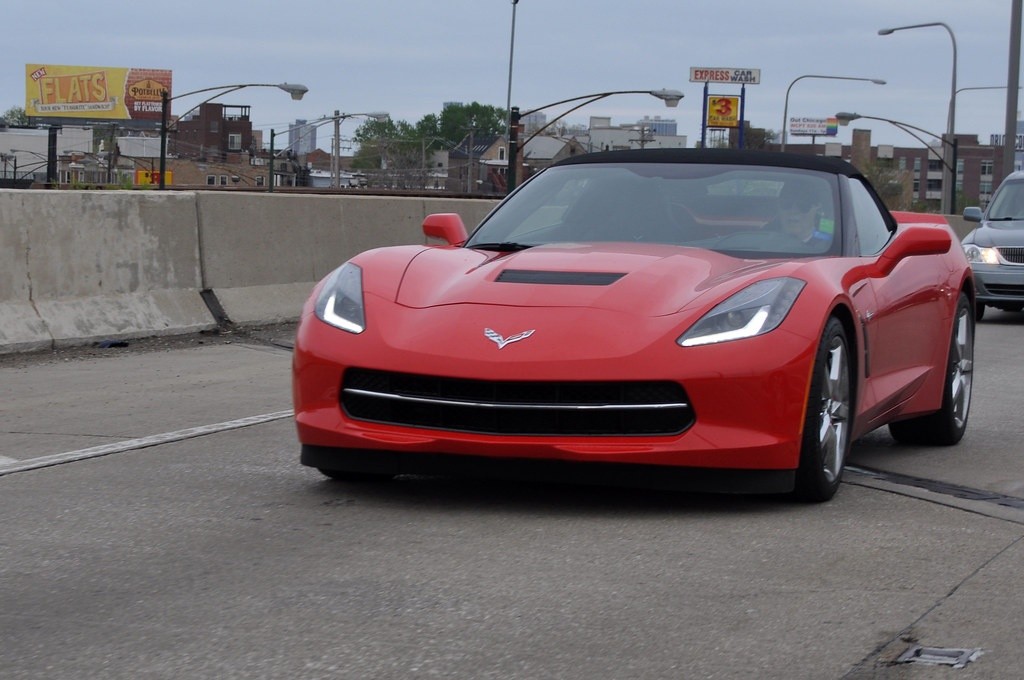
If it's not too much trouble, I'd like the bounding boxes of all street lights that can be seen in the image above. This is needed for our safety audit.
[832,111,958,214]
[158,83,310,190]
[876,21,957,135]
[504,87,685,196]
[267,111,390,193]
[781,74,888,153]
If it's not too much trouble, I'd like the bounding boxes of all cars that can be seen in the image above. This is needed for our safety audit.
[959,171,1024,321]
[290,146,979,505]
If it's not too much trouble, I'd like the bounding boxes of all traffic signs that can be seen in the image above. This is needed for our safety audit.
[688,66,761,85]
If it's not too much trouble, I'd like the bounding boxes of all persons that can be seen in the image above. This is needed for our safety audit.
[760,175,834,256]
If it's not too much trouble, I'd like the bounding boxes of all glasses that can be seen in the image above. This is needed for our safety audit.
[778,199,813,209]
